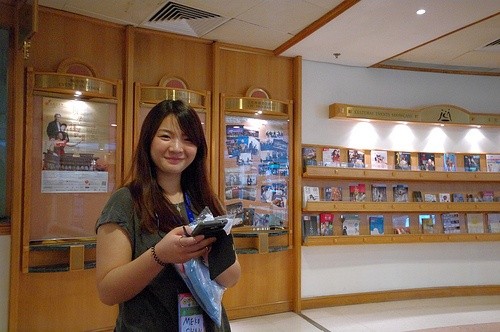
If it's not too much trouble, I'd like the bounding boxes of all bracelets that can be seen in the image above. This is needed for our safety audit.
[164,191,183,218]
[152,244,165,266]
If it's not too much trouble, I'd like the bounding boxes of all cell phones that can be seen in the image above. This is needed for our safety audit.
[192,219,228,241]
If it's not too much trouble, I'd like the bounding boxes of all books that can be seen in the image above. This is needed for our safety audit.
[302,148,316,167]
[348,150,365,169]
[441,213,500,234]
[486,155,500,172]
[418,152,435,171]
[443,153,456,172]
[453,191,494,202]
[371,151,387,169]
[391,214,436,235]
[371,184,450,203]
[322,148,341,167]
[342,214,384,235]
[303,213,333,236]
[464,155,480,172]
[303,183,367,208]
[394,151,410,171]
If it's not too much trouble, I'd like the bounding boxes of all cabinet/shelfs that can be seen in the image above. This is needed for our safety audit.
[301,145,500,247]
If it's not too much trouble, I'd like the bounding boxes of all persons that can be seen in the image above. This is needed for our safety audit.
[46,114,69,170]
[226,125,288,226]
[95,99,241,332]
